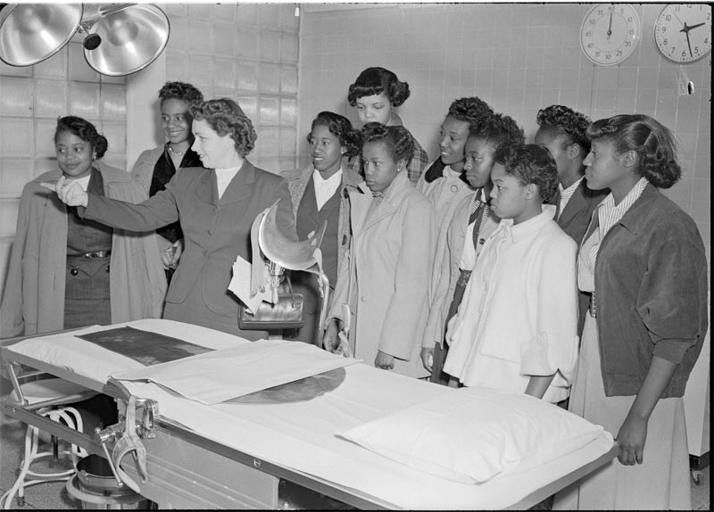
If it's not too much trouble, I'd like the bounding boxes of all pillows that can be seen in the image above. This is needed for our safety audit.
[333,385,606,487]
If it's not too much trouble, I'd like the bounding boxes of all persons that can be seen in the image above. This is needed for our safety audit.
[0,114,167,342]
[128,80,202,294]
[39,98,296,342]
[550,114,708,511]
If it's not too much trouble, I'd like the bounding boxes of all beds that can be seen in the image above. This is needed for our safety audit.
[0,317,619,510]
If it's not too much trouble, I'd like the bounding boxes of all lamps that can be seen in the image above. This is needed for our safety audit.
[0,4,173,80]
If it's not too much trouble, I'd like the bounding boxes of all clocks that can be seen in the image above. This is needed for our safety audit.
[576,3,643,68]
[651,2,713,64]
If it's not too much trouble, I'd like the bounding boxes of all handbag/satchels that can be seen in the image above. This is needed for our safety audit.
[234,292,306,331]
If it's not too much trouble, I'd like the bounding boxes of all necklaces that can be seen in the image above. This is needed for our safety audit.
[167,145,191,156]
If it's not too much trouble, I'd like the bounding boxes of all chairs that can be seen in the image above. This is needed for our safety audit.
[0,335,103,504]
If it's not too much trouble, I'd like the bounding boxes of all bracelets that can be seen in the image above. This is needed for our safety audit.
[81,190,86,206]
